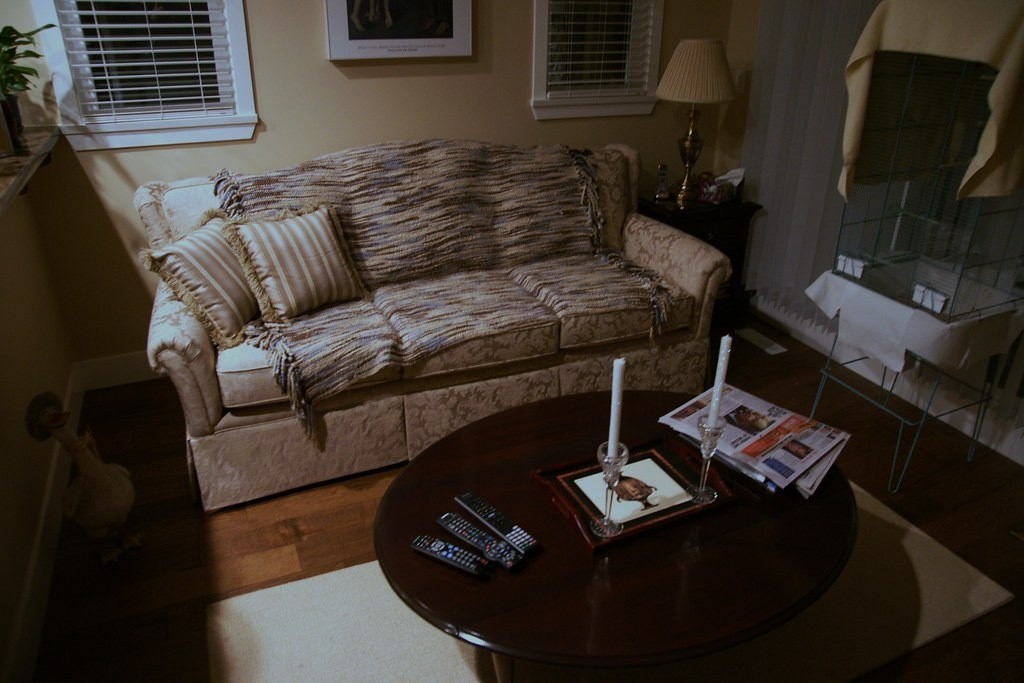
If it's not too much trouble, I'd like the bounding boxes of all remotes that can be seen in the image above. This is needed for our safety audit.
[454,488,540,555]
[436,511,524,572]
[409,534,490,575]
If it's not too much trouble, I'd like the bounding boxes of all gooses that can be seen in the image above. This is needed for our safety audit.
[37,403,142,564]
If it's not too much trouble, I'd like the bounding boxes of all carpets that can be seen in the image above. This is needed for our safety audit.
[206,479,1014,683]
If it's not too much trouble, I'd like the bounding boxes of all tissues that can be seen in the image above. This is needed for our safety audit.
[695,167,747,204]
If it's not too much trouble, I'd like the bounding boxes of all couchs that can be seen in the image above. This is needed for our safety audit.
[133,138,729,513]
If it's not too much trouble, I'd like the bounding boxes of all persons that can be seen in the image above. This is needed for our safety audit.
[350,0,451,35]
[724,412,770,431]
[784,440,809,458]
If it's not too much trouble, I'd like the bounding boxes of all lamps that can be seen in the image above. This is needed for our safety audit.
[654,37,737,209]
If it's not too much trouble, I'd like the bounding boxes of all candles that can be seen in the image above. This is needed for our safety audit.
[607,357,627,457]
[709,335,732,427]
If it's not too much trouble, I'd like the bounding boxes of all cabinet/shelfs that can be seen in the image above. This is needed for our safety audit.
[639,192,763,316]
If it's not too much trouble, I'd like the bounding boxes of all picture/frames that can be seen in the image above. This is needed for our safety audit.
[324,0,473,61]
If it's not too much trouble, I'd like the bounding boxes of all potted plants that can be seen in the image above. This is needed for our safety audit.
[0,23,55,159]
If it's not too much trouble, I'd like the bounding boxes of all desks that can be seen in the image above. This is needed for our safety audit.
[804,258,1024,496]
[0,126,59,215]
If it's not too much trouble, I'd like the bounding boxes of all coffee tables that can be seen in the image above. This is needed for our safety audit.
[372,390,859,683]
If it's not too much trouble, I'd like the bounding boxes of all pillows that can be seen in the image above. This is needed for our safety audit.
[137,208,261,350]
[222,202,369,326]
